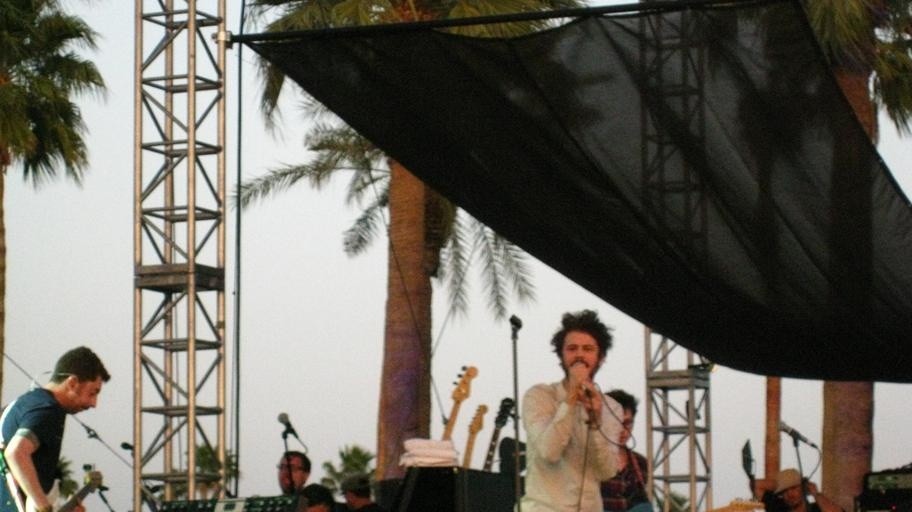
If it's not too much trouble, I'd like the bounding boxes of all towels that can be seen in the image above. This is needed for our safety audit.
[399,439,458,468]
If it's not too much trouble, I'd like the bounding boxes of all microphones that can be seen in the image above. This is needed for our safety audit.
[776,420,818,449]
[278,413,299,439]
[121,443,133,450]
[571,359,591,400]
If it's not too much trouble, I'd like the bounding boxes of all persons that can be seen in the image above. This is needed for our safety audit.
[298,483,337,512]
[339,472,380,511]
[276,450,311,494]
[512,309,625,511]
[748,469,848,511]
[603,388,654,511]
[1,346,113,512]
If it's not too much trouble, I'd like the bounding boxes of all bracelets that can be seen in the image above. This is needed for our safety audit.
[814,492,822,499]
[35,504,53,512]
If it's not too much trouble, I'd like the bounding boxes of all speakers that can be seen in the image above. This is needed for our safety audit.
[388,467,526,512]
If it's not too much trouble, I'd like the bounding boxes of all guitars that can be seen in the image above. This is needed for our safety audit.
[481,397,515,473]
[26,472,104,512]
[443,365,477,442]
[463,405,489,470]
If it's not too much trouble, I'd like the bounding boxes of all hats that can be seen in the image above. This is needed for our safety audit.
[775,468,801,494]
